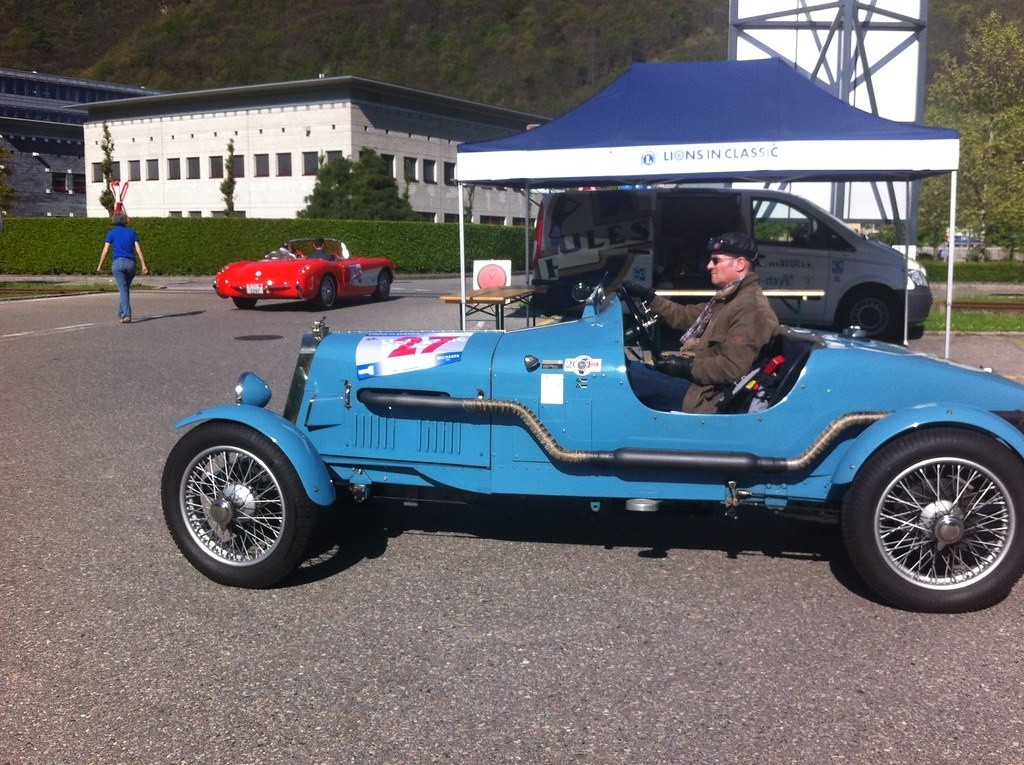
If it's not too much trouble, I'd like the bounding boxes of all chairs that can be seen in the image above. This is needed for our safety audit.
[715,324,816,414]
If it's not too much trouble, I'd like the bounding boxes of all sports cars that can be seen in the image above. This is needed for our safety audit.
[213,237,395,309]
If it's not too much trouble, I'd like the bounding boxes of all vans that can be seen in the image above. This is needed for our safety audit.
[532,187,934,340]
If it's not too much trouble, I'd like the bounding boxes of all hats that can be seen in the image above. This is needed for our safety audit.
[708,232,761,267]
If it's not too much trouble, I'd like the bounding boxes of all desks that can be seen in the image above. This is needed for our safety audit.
[440,285,536,330]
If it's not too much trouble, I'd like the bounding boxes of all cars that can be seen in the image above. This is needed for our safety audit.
[946,236,982,248]
[159,271,1024,613]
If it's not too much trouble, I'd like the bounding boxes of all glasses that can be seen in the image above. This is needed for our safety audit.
[707,257,736,266]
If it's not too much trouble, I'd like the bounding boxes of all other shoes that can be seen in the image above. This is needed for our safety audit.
[119,316,131,323]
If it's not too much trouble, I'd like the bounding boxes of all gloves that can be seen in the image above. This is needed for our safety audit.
[651,355,694,378]
[618,280,655,307]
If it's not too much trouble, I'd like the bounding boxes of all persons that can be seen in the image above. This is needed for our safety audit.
[282,238,338,260]
[622,233,778,414]
[97,212,149,323]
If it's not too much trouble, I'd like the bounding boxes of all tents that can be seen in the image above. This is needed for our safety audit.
[947,235,981,250]
[456,56,961,359]
